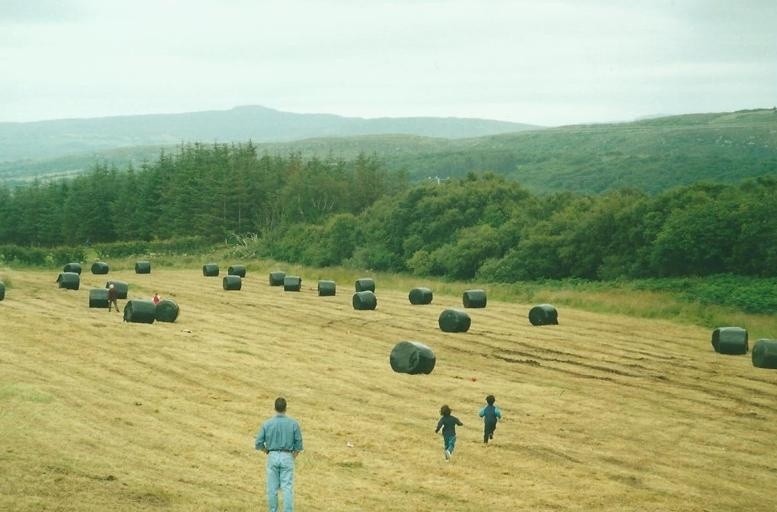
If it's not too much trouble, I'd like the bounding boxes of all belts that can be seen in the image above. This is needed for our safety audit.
[267,449,294,454]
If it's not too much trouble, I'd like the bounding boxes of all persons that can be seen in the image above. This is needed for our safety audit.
[434,405,463,462]
[253,397,304,511]
[479,394,501,444]
[108,284,119,312]
[152,292,159,304]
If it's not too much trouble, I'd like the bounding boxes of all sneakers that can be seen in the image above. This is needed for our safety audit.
[445,449,452,463]
[482,435,493,448]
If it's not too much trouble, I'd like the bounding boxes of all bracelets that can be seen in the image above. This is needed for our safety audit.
[265,450,268,454]
